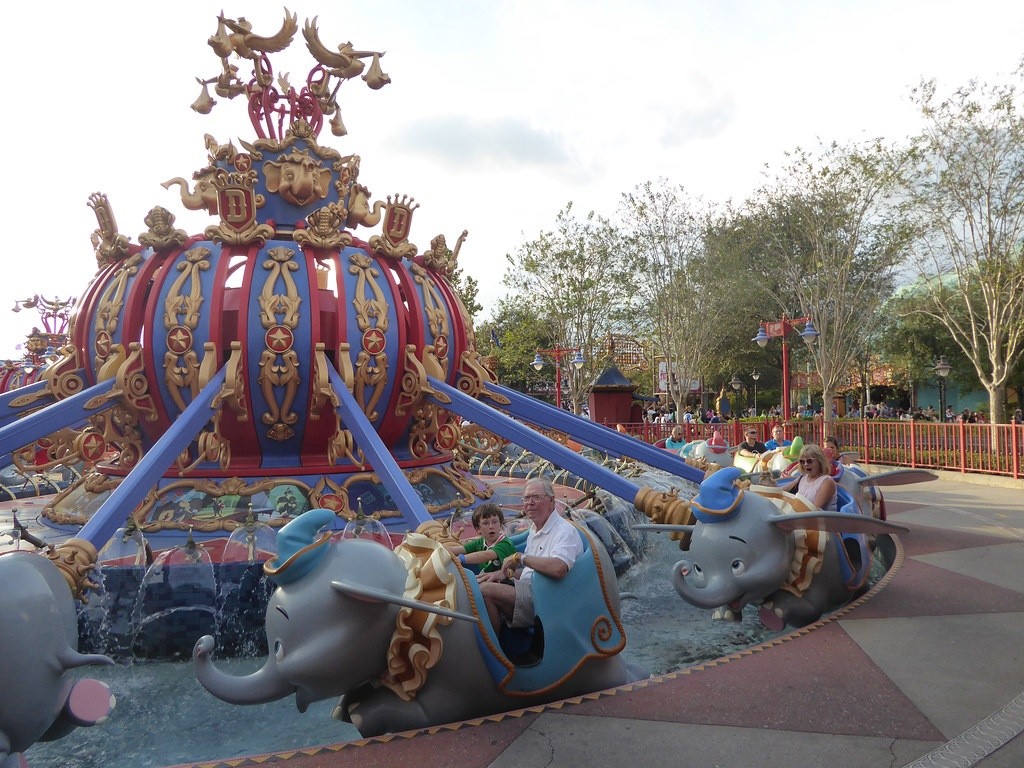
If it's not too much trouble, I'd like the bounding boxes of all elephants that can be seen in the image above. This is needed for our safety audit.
[676,431,740,467]
[192,509,640,739]
[670,468,911,633]
[0,551,117,768]
[738,452,939,554]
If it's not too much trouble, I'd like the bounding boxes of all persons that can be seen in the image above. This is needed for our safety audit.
[449,402,1024,642]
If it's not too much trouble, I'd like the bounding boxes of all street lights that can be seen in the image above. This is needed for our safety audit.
[749,365,762,417]
[529,345,586,408]
[933,354,953,423]
[728,373,744,417]
[665,372,679,413]
[751,311,821,441]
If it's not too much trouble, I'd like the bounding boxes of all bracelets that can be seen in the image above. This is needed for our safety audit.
[753,453,756,458]
[513,551,521,564]
[459,554,466,566]
[521,554,528,565]
[508,568,513,577]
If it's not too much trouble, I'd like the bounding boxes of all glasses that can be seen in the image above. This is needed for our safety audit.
[800,458,818,465]
[521,495,550,502]
[677,432,682,435]
[478,521,501,529]
[748,431,757,434]
